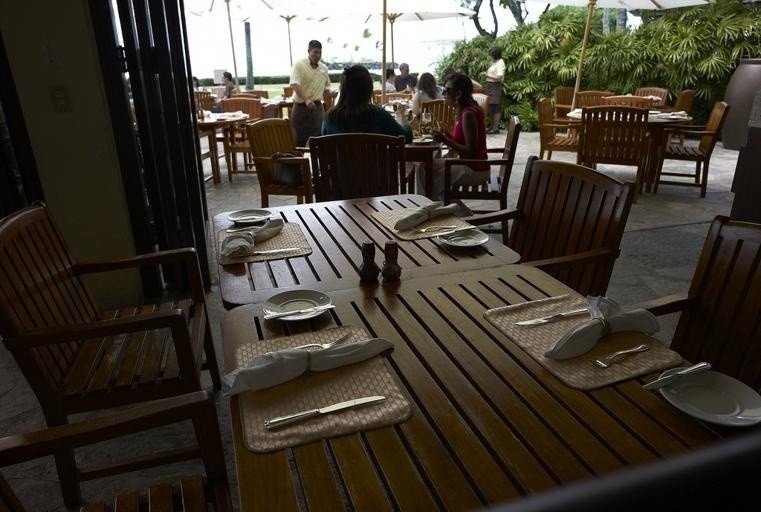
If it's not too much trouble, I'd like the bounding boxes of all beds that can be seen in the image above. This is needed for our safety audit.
[184,0,276,89]
[542,0,712,138]
[346,0,477,82]
[249,0,351,65]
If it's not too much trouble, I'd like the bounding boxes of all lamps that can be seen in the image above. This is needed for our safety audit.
[626,217,761,511]
[0,194,225,512]
[467,157,638,298]
[0,391,228,512]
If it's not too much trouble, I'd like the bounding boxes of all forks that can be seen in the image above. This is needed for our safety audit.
[589,344,649,369]
[264,331,352,354]
[407,224,456,232]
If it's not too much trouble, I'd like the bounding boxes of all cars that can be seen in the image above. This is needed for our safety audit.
[481,72,486,81]
[381,96,441,144]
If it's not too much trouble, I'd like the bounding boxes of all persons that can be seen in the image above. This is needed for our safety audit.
[192,77,204,92]
[417,72,490,201]
[321,65,413,196]
[386,63,482,121]
[480,47,505,135]
[289,40,338,146]
[212,72,235,113]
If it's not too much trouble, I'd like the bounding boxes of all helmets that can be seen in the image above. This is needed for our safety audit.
[438,228,490,249]
[225,208,271,224]
[263,289,332,321]
[649,110,688,120]
[657,366,761,427]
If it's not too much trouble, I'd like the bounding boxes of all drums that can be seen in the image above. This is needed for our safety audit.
[515,308,588,328]
[262,394,385,434]
[428,225,475,238]
[638,361,712,390]
[229,248,302,258]
[229,215,275,221]
[261,302,334,320]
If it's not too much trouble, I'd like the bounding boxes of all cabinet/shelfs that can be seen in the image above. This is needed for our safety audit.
[196,109,199,120]
[199,110,204,120]
[380,240,402,282]
[356,240,379,282]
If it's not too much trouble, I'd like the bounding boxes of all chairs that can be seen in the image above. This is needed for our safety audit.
[467,157,638,298]
[487,126,500,134]
[195,81,729,245]
[0,194,225,512]
[0,391,228,512]
[626,217,761,511]
